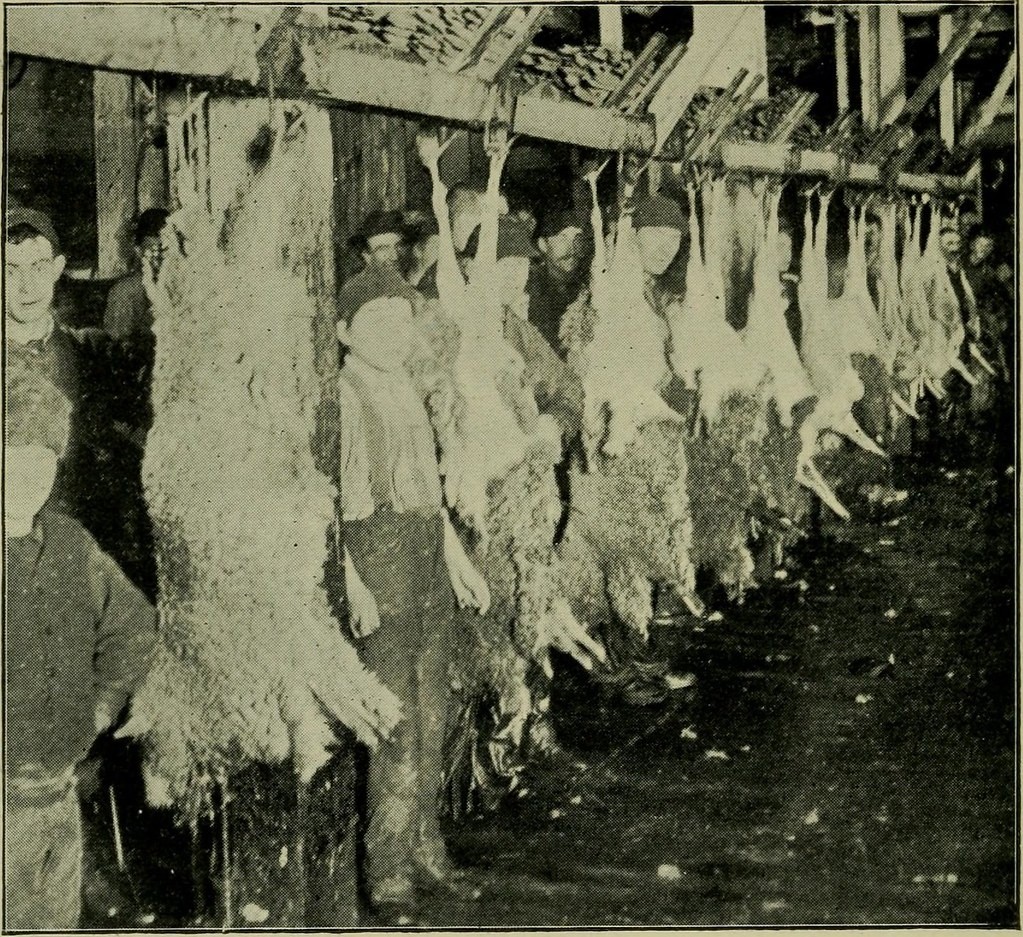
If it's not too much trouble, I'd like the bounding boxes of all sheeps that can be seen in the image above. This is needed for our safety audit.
[114,93,997,810]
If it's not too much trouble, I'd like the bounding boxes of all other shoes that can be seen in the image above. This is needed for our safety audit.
[620,682,670,709]
[428,877,482,902]
[378,901,418,926]
[660,671,696,689]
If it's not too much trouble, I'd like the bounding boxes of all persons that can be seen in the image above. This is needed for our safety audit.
[6,132,1022,928]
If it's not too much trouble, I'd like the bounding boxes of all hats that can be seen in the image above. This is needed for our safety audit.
[337,268,417,326]
[8,208,60,249]
[632,197,687,230]
[464,218,540,258]
[362,211,404,238]
[7,374,73,455]
